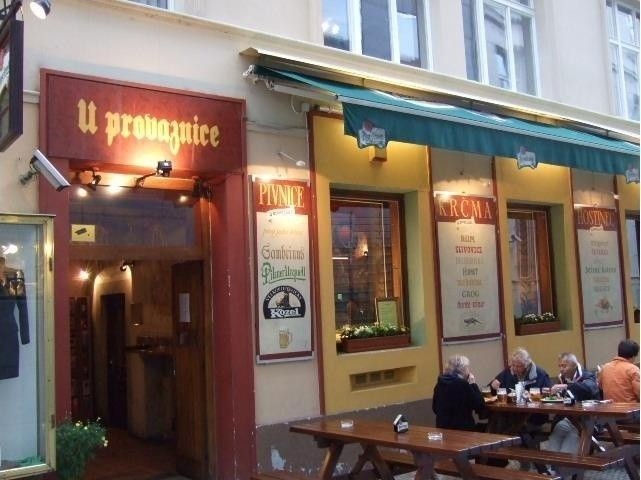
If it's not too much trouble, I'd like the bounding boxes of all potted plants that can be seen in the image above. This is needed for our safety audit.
[55,415,110,480]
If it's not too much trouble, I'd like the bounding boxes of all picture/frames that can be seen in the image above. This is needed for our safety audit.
[376,298,401,326]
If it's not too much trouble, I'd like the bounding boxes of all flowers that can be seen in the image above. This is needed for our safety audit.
[340,321,404,339]
[521,312,558,324]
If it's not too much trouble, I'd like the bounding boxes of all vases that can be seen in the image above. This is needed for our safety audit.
[340,333,411,353]
[515,320,562,336]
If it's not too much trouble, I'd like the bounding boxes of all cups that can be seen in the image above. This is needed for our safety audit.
[280,329,293,347]
[542,388,551,397]
[483,385,492,401]
[530,388,541,404]
[498,388,507,404]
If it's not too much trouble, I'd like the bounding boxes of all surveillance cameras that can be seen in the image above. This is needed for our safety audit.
[30,148,73,194]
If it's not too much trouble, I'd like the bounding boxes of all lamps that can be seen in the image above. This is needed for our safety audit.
[82,160,213,207]
[29,0,52,21]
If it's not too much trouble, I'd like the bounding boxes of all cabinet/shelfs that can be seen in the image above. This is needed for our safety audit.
[0,212,57,479]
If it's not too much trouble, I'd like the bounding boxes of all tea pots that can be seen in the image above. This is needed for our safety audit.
[512,382,528,406]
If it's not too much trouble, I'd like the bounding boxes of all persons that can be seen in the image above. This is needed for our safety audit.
[431,352,512,470]
[488,344,553,447]
[597,337,640,465]
[540,351,602,480]
[334,289,375,331]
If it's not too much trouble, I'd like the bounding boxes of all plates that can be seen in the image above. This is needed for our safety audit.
[542,397,565,403]
